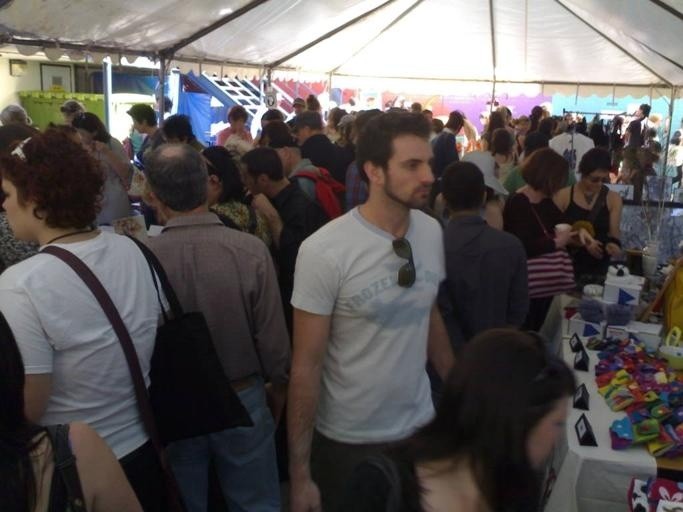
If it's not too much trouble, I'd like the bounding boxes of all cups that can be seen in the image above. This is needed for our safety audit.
[555,224,572,233]
[674,188,683,203]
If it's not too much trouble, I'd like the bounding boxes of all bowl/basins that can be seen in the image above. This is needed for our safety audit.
[656,345,682,372]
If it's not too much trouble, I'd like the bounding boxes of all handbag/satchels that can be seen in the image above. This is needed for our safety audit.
[150,312,254,440]
[527,251,576,300]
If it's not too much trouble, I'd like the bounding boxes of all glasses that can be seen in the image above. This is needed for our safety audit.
[393,238,415,287]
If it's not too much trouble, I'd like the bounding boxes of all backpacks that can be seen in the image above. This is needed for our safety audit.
[297,167,345,219]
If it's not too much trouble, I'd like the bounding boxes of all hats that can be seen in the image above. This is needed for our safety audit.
[258,95,321,149]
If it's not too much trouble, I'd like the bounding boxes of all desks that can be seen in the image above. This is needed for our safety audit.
[613,201,682,265]
[535,291,683,512]
